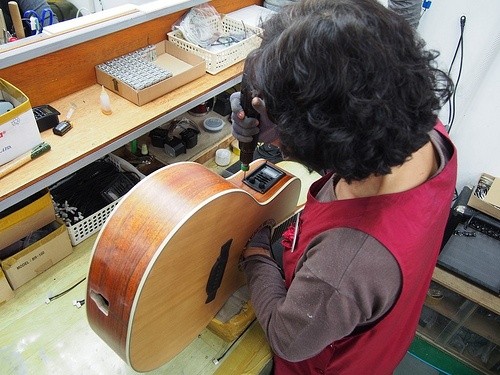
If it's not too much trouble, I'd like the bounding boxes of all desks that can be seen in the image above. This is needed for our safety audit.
[0,0,300,375]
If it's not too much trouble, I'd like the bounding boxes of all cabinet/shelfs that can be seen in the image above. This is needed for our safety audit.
[416,263,500,375]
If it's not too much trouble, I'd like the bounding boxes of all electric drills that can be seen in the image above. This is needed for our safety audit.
[240,50,266,180]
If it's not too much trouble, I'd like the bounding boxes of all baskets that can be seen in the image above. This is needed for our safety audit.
[167,13,265,76]
[49,152,147,247]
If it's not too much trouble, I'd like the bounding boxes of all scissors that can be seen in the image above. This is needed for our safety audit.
[25,9,53,33]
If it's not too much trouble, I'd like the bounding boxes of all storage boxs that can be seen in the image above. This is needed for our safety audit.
[167,12,264,75]
[50,153,146,246]
[0,77,41,166]
[95,40,206,107]
[468,173,500,220]
[0,187,73,304]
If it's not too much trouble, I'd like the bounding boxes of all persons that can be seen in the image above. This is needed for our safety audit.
[230,0,457,375]
[0,0,60,35]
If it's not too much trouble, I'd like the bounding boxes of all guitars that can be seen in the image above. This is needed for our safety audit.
[85,159,324,373]
